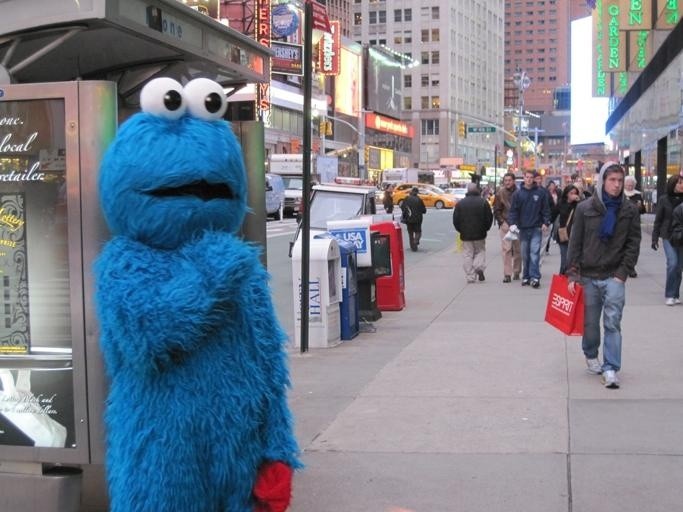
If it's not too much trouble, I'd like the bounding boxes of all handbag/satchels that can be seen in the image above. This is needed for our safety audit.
[544,273,586,338]
[556,227,569,243]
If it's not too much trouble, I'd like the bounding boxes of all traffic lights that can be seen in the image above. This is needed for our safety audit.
[459,121,464,137]
[326,122,332,135]
[365,147,370,162]
[318,121,325,135]
[539,168,547,176]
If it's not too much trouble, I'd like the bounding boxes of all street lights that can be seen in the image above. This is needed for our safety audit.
[559,119,568,174]
[510,67,531,177]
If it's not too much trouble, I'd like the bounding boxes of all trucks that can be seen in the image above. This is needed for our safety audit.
[266,151,339,219]
[376,164,435,193]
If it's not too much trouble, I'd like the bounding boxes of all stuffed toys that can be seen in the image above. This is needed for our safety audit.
[90,76,304,511]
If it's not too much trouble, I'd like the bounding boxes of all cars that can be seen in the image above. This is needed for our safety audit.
[374,190,387,204]
[288,176,376,260]
[390,181,468,209]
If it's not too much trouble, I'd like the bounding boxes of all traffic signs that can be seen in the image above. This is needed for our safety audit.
[467,126,496,133]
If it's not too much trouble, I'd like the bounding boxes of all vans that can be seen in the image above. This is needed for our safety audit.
[265,172,287,221]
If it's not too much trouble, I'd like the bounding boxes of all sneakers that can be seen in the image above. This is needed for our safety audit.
[502,275,511,283]
[521,278,529,287]
[600,370,620,389]
[476,269,485,281]
[529,278,538,288]
[664,297,681,306]
[512,271,519,281]
[583,355,602,375]
